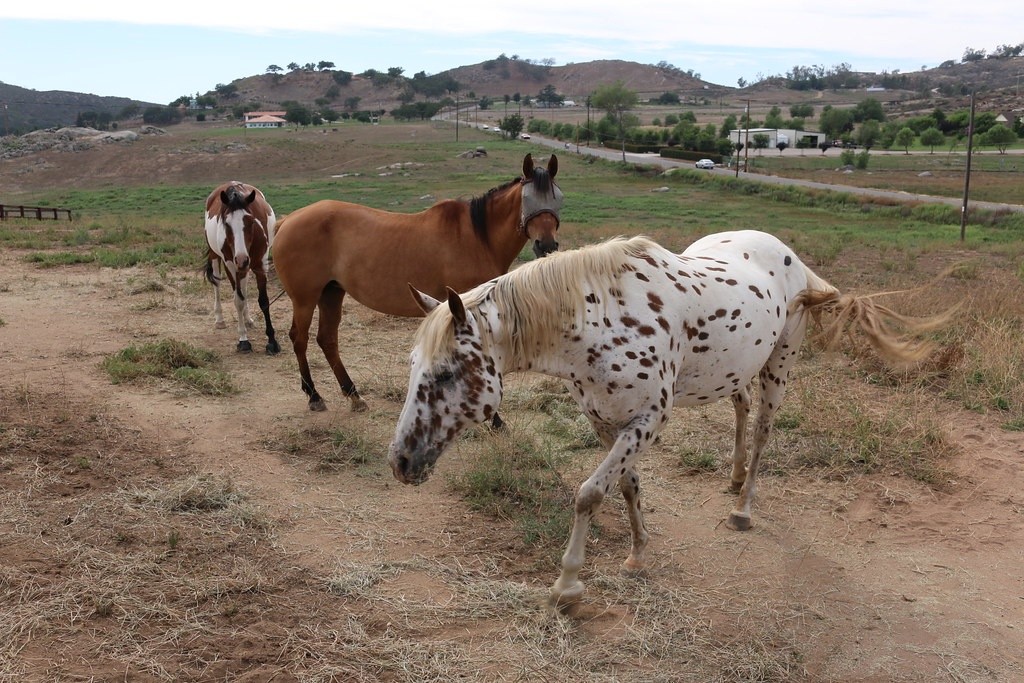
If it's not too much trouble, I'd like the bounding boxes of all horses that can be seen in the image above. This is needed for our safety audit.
[197,181,283,357]
[271,153,566,413]
[387,230,974,620]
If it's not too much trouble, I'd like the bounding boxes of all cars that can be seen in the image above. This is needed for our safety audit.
[695,158,716,170]
[482,123,489,129]
[493,127,501,132]
[521,134,530,139]
